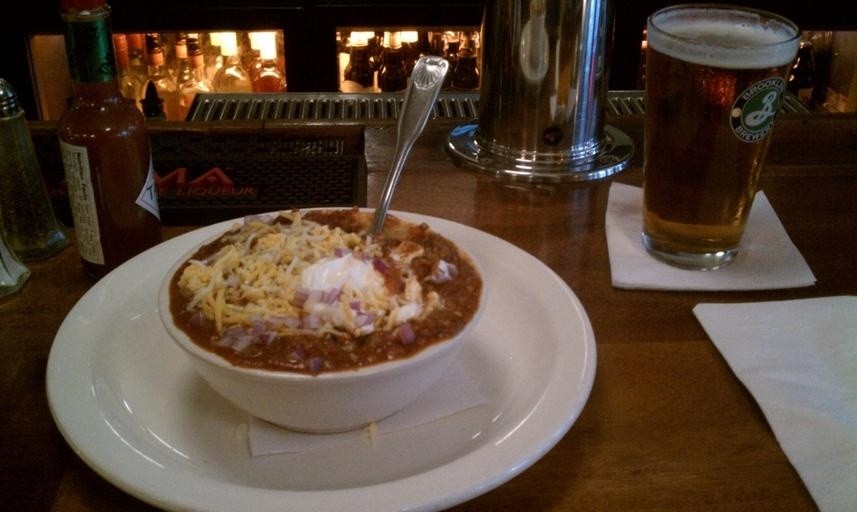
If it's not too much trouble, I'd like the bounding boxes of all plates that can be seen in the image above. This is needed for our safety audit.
[44,206,599,512]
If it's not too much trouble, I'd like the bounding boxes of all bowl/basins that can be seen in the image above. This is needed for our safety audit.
[157,209,489,434]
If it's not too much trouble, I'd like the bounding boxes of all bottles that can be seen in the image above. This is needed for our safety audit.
[114,33,289,120]
[56,2,162,285]
[788,41,813,103]
[1,78,71,263]
[336,29,481,94]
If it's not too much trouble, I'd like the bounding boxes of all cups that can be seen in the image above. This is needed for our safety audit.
[640,2,804,271]
